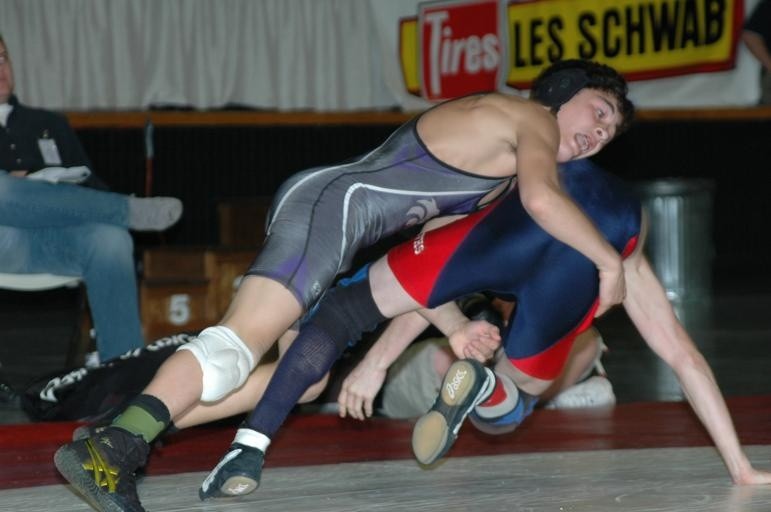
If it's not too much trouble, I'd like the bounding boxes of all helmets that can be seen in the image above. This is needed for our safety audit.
[529,57,629,106]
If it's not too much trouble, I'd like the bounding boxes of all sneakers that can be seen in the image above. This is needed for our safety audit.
[551,375,615,410]
[199,441,266,499]
[413,358,498,466]
[54,421,150,512]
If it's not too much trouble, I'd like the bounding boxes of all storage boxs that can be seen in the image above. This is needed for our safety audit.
[217,194,273,252]
[140,242,219,344]
[217,250,260,323]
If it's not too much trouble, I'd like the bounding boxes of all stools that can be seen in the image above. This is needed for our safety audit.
[1,271,95,389]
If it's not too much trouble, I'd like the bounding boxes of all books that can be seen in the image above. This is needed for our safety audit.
[25,166,92,185]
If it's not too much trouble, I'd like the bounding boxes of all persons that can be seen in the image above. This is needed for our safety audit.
[743,1,771,105]
[26,305,622,457]
[49,62,651,511]
[0,35,184,357]
[193,153,771,502]
